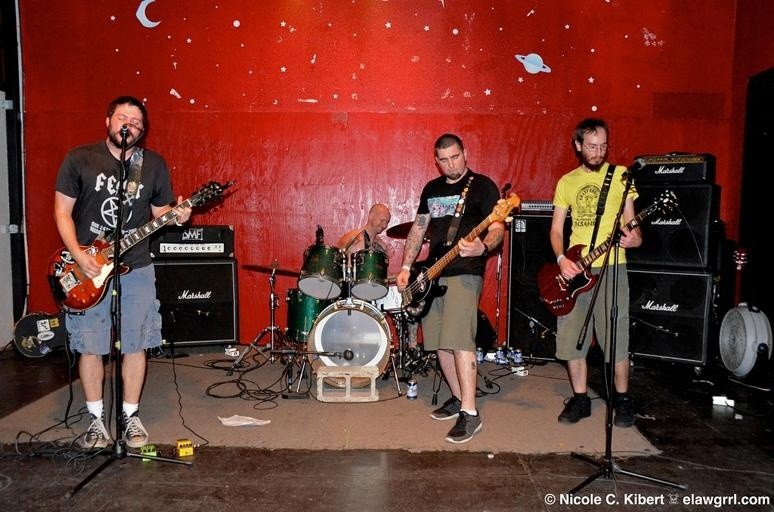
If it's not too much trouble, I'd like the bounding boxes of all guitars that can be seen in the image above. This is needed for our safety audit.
[402,192,522,319]
[47,182,235,313]
[537,191,679,317]
[13,310,69,359]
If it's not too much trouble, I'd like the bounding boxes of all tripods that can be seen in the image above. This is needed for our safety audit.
[226,300,295,374]
[566,243,687,497]
[383,318,428,382]
[69,206,193,492]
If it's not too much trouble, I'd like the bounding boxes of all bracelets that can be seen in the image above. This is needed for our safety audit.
[556,254,565,265]
[174,216,182,228]
[401,264,411,270]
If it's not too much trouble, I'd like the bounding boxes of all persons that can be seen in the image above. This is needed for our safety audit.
[336,203,395,270]
[549,118,643,428]
[52,95,192,451]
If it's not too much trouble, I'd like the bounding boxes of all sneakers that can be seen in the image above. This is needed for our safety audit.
[558,395,591,424]
[614,398,635,428]
[430,395,462,421]
[84,412,110,448]
[445,408,482,444]
[122,409,149,448]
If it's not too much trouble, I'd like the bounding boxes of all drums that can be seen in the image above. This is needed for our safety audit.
[298,245,347,300]
[306,299,395,388]
[351,249,388,301]
[286,287,321,343]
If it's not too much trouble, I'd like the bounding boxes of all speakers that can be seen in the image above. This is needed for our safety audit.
[508,212,581,364]
[626,186,719,270]
[148,261,241,347]
[618,268,716,372]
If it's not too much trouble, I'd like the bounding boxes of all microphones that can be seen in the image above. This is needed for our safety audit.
[621,158,647,178]
[502,183,512,193]
[121,124,130,139]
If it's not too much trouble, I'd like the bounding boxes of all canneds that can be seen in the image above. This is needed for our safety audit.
[406,379,418,399]
[476,346,523,365]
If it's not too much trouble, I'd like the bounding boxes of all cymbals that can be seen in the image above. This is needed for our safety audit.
[385,222,433,240]
[242,264,299,278]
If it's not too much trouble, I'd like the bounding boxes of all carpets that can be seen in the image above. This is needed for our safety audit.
[0,339,664,457]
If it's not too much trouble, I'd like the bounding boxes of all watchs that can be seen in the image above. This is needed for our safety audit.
[396,134,506,444]
[480,244,489,257]
